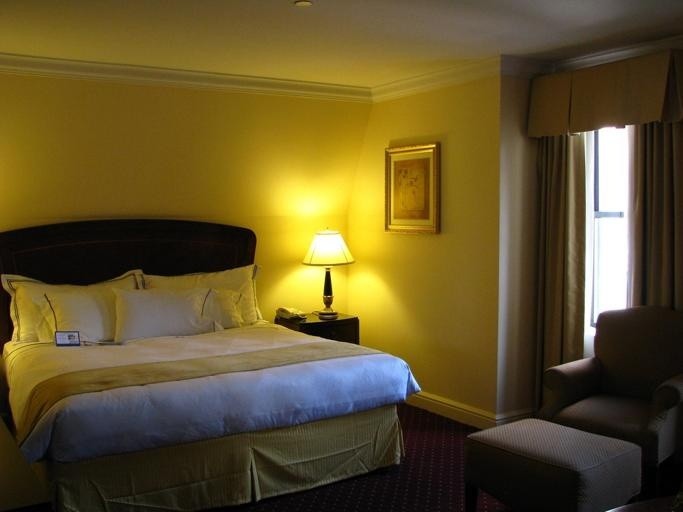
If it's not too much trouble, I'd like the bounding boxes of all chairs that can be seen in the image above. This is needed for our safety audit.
[533,303,682,499]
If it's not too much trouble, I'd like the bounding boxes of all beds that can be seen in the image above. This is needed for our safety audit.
[0,217,413,512]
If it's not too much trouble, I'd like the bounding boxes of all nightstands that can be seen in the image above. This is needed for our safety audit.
[273,308,360,350]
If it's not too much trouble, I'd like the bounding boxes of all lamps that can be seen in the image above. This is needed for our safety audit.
[299,229,357,323]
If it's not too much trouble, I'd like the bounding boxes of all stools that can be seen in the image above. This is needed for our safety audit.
[462,414,644,512]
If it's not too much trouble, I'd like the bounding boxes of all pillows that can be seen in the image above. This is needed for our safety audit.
[0,262,261,344]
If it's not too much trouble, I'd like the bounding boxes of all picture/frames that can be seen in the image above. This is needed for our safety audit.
[382,139,442,234]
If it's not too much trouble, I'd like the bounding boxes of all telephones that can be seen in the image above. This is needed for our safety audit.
[276,307,305,320]
